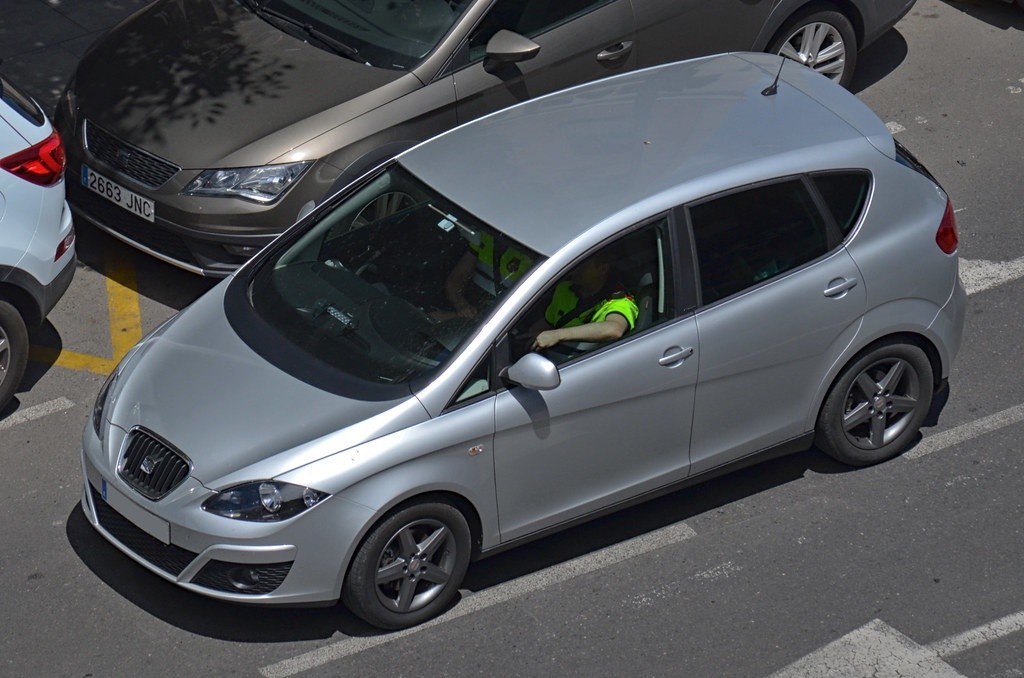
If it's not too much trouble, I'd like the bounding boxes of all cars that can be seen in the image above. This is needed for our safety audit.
[80,51,966,634]
[53,0,918,275]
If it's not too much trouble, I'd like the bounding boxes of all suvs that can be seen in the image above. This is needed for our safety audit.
[0,75,77,415]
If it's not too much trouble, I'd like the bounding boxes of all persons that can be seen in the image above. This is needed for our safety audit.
[516,248,640,367]
[424,226,533,323]
